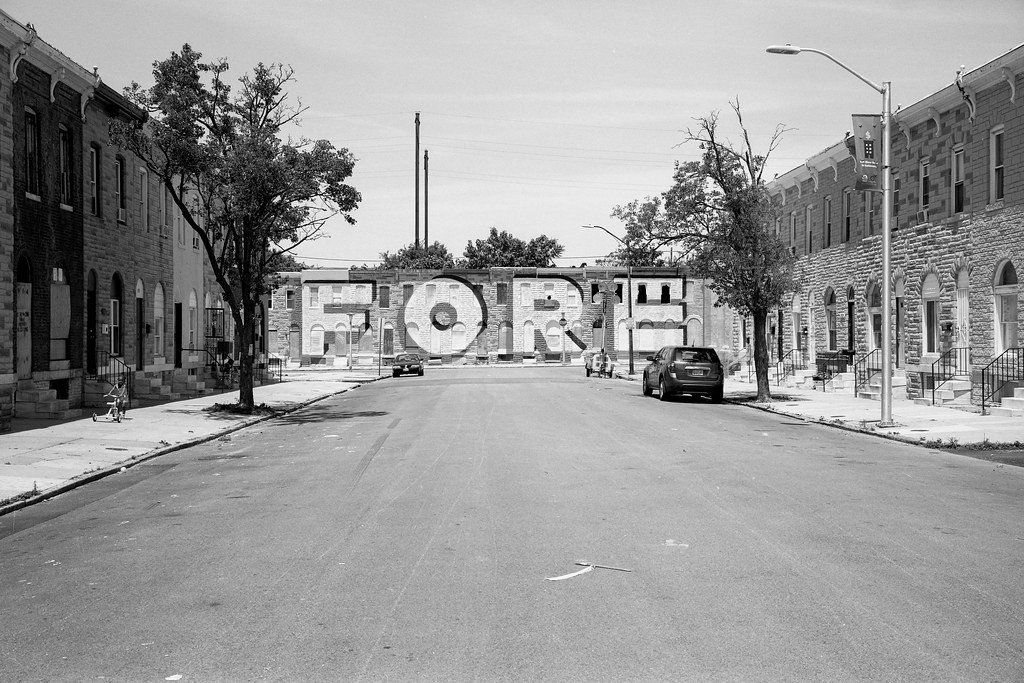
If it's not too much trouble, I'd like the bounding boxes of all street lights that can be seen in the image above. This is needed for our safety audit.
[765,43,900,429]
[581,224,636,376]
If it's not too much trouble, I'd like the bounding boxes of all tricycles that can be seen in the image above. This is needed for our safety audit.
[92,394,126,423]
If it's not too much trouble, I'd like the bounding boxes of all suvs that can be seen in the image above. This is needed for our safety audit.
[642,344,725,404]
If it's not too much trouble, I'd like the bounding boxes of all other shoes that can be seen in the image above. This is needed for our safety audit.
[122,414,126,418]
[118,414,121,418]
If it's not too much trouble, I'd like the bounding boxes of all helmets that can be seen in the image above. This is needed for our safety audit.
[118,376,124,383]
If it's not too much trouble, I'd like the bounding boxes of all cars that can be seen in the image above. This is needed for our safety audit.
[391,353,424,377]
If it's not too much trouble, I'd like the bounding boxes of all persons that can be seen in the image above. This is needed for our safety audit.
[108,375,129,419]
[600,353,610,379]
